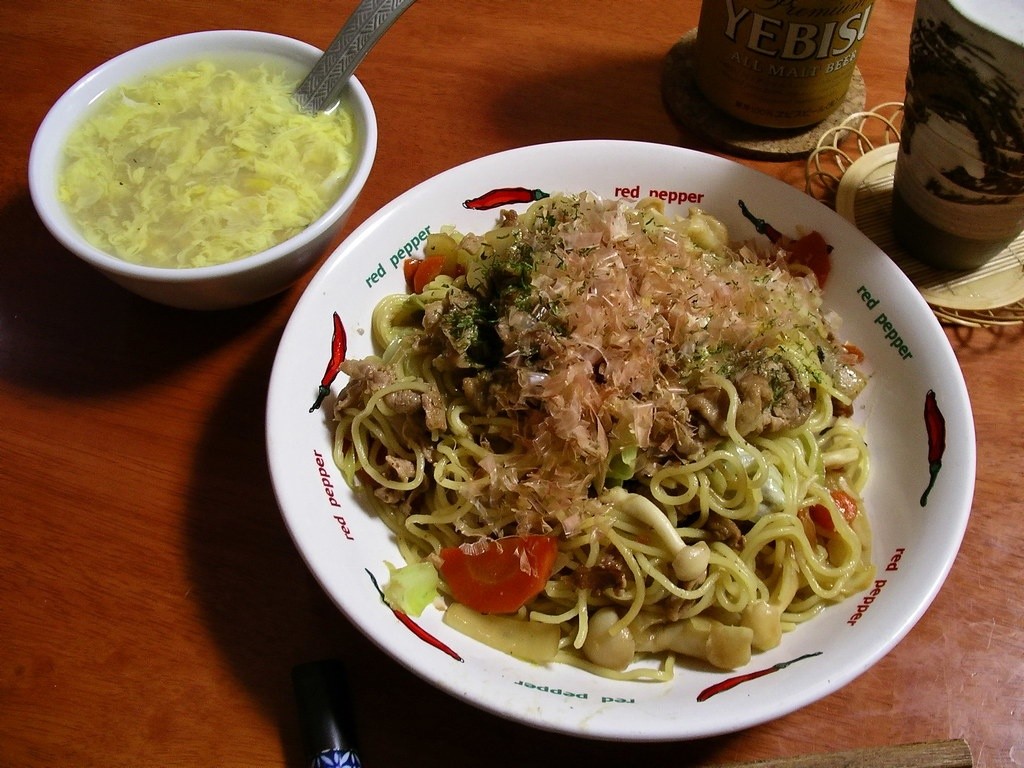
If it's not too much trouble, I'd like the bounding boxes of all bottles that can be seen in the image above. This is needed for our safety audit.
[288,655,364,767]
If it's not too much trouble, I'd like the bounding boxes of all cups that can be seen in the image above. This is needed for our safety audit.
[894,0,1024,269]
[696,0,872,128]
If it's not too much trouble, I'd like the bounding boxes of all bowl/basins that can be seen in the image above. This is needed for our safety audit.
[28,30,378,312]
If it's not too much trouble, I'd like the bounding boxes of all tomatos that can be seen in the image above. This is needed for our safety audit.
[442,532,557,612]
[414,257,446,292]
[788,231,829,288]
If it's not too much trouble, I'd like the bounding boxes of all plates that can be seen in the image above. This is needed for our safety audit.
[267,138,978,742]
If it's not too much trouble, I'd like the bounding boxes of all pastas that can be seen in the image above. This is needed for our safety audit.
[334,197,873,682]
